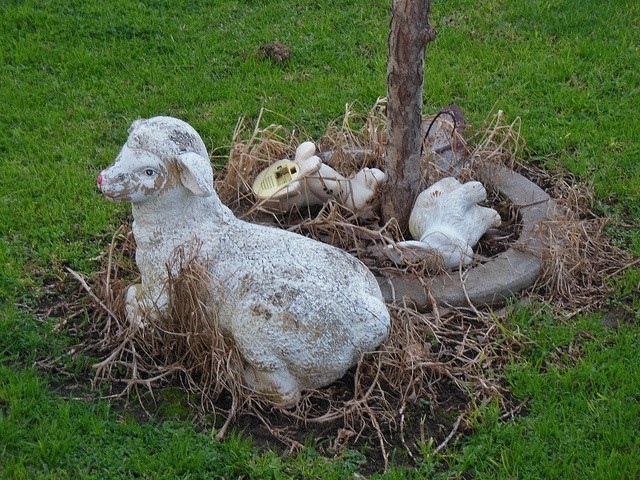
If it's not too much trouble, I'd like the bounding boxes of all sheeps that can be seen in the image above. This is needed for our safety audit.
[95,114,394,410]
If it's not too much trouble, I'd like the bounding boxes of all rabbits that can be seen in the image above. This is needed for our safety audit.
[383,174,504,272]
